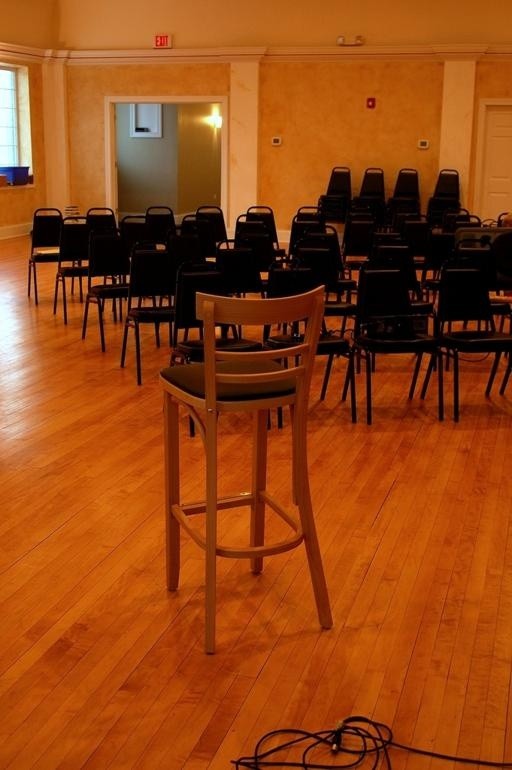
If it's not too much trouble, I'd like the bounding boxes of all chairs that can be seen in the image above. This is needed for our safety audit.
[159,285,333,655]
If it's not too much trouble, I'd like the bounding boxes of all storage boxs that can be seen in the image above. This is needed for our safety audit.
[0,167,29,185]
